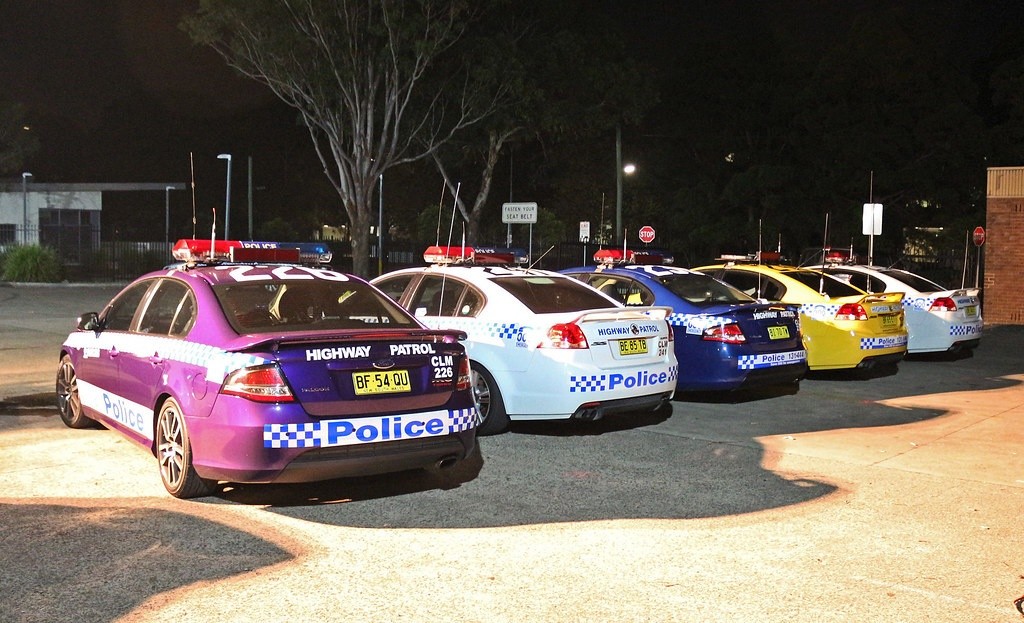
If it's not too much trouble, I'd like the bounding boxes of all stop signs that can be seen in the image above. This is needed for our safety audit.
[639,226,656,243]
[972,227,985,247]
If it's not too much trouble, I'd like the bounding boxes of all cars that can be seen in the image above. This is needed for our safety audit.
[318,245,680,436]
[622,252,908,380]
[56,239,476,499]
[739,252,983,360]
[535,250,809,401]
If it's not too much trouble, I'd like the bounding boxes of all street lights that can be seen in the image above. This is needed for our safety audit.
[217,153,230,238]
[616,163,635,247]
[22,172,33,244]
[371,158,383,275]
